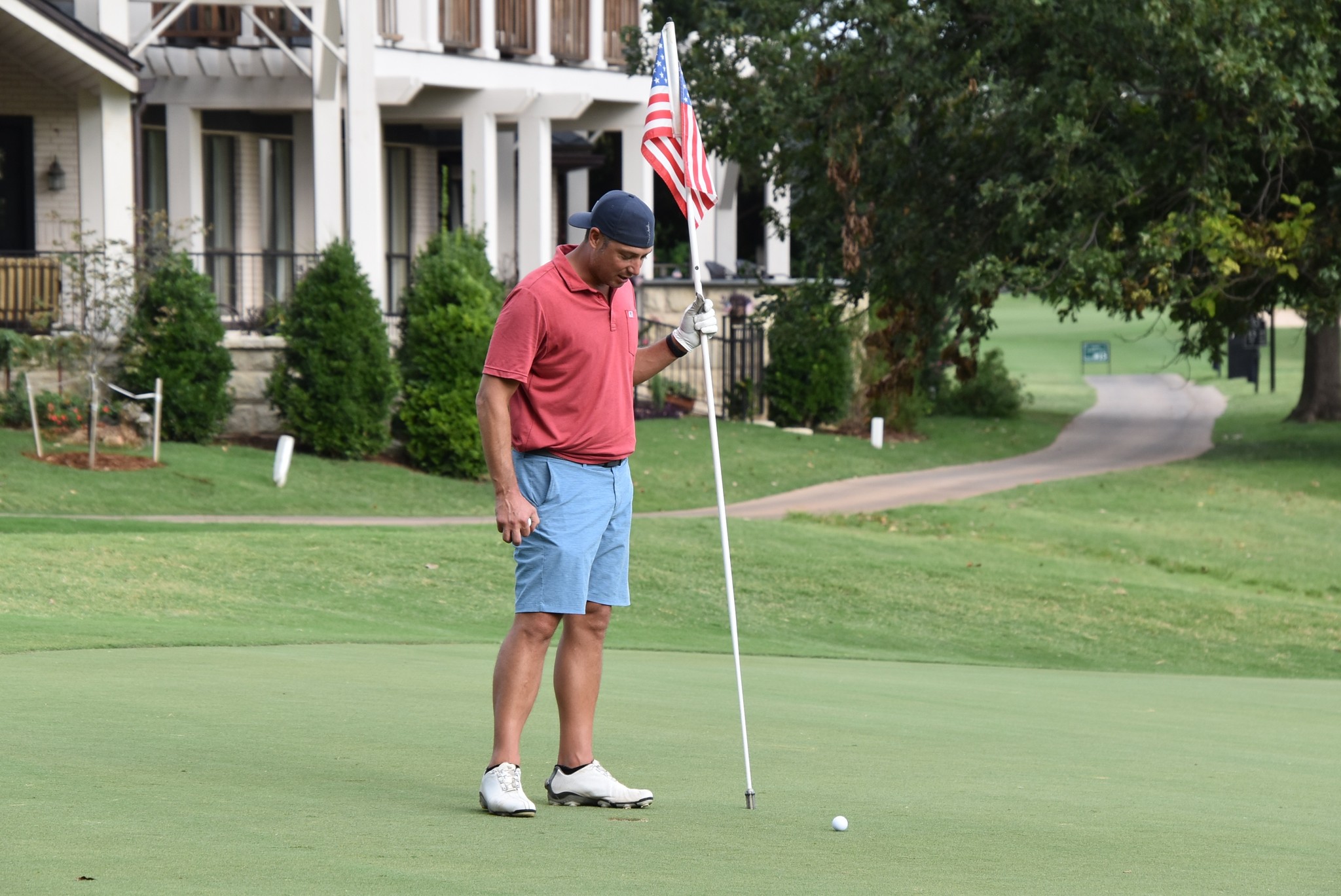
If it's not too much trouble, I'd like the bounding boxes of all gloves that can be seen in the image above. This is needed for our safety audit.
[673,293,718,352]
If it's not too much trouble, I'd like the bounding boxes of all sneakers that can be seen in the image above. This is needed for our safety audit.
[544,758,653,809]
[479,762,536,817]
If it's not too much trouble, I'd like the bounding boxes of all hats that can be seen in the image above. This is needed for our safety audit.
[568,190,655,247]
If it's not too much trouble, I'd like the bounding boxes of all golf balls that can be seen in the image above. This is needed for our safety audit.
[832,816,848,831]
[528,517,532,527]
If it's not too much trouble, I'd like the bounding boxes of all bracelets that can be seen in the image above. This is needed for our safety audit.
[666,333,688,358]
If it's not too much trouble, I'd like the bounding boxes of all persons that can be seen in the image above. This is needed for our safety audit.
[474,190,720,816]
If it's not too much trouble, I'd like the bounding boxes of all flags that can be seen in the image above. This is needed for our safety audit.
[641,21,718,227]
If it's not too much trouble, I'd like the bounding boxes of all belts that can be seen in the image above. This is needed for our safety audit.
[527,449,625,466]
